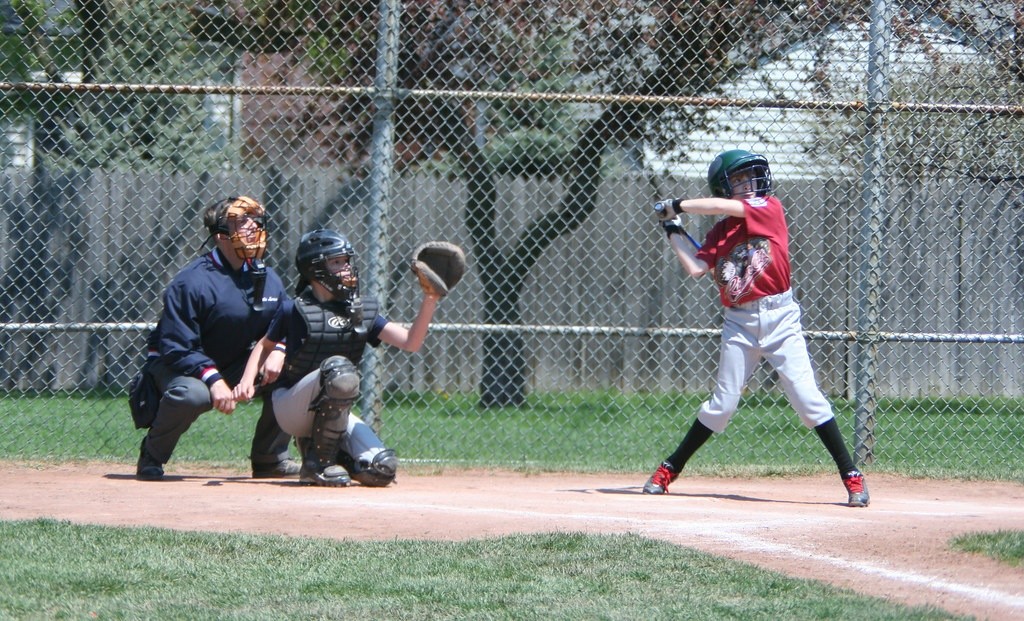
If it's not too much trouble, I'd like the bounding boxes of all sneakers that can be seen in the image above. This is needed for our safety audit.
[252,457,302,479]
[298,462,352,487]
[644,458,680,494]
[842,469,869,507]
[136,435,164,480]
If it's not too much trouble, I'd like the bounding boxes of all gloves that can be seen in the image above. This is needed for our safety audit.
[658,212,682,238]
[656,197,687,221]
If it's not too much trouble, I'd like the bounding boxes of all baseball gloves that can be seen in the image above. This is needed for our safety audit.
[411,239,466,299]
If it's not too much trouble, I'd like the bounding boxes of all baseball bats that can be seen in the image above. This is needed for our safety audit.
[654,202,704,252]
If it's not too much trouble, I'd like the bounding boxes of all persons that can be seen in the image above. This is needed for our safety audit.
[232,230,465,487]
[643,150,870,507]
[137,196,301,478]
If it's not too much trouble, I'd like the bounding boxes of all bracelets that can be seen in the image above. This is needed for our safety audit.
[672,199,684,214]
[667,225,681,239]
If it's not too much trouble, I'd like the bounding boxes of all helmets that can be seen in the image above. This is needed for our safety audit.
[707,150,773,199]
[295,229,360,300]
